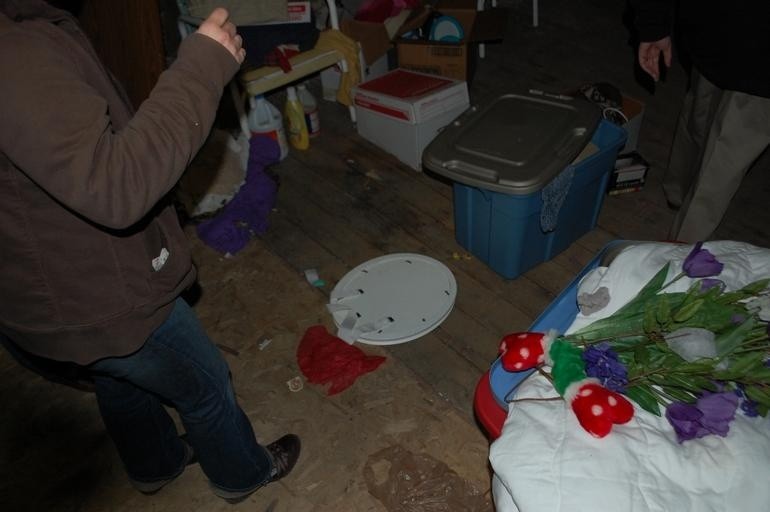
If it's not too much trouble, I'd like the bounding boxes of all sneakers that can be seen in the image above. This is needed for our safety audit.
[138,432,200,495]
[219,432,302,504]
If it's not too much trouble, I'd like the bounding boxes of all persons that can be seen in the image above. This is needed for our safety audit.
[1,1,303,506]
[635,2,769,245]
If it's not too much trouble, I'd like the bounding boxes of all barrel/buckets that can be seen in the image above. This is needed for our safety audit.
[297,84,320,135]
[247,96,289,163]
[284,87,310,150]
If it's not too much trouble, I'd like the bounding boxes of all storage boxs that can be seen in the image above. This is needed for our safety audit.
[420,86,628,280]
[351,10,480,174]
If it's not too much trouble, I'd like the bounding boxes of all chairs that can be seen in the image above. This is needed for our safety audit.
[177,0,357,139]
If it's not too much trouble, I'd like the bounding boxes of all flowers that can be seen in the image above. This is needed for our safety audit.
[500,241,770,444]
